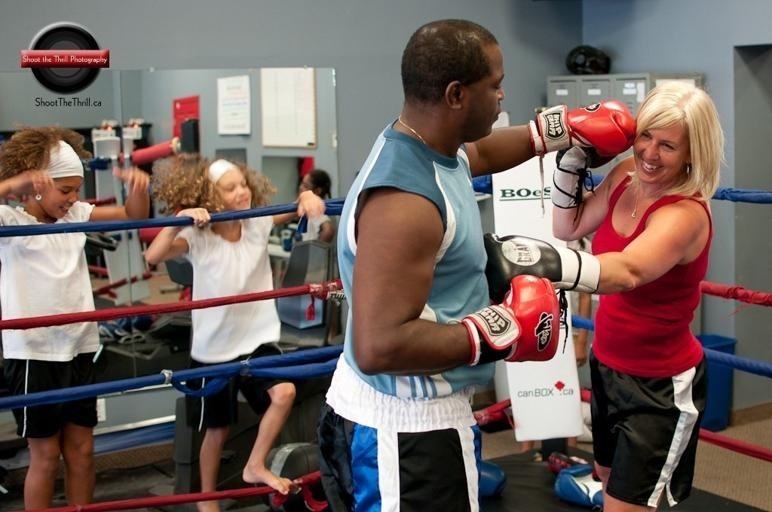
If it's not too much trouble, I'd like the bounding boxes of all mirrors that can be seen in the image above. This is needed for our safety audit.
[0,64,337,392]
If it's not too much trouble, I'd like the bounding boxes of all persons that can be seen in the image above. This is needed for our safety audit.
[314,19,636,512]
[0,124,151,511]
[519,237,591,452]
[144,153,326,512]
[291,170,333,249]
[483,83,727,512]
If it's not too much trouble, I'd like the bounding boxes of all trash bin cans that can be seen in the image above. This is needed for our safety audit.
[695,334,738,431]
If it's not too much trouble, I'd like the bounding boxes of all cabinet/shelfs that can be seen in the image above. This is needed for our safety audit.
[572,297,596,389]
[615,79,647,162]
[549,80,578,106]
[580,78,611,174]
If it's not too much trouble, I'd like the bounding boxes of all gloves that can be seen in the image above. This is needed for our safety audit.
[551,146,617,208]
[461,273,560,368]
[483,232,600,305]
[528,99,636,158]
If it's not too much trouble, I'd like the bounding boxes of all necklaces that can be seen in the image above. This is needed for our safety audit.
[632,190,639,218]
[399,116,426,145]
